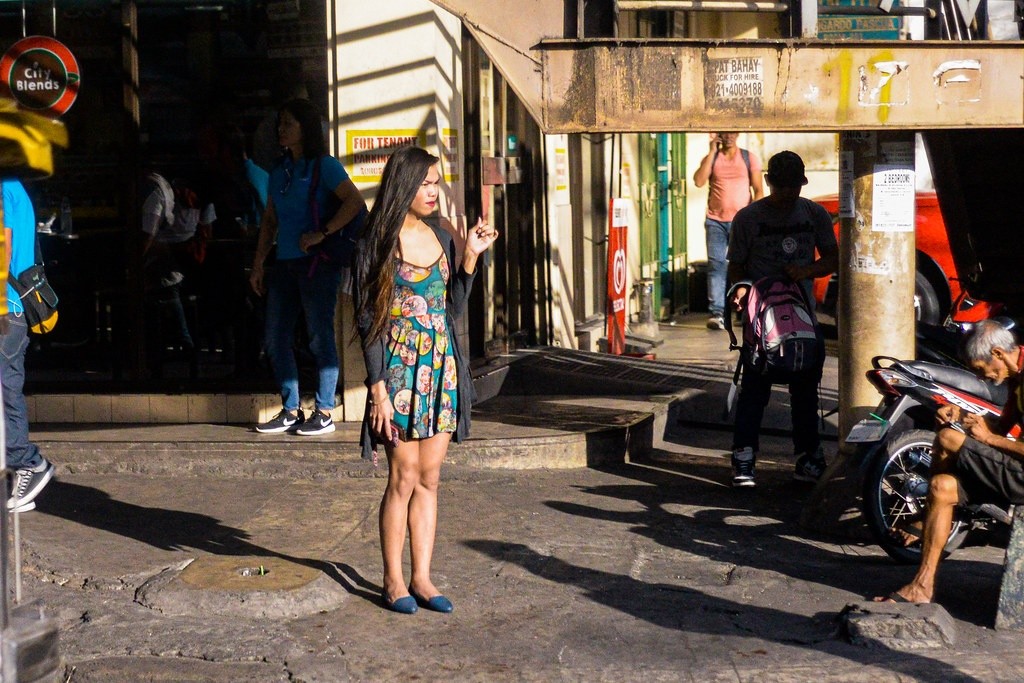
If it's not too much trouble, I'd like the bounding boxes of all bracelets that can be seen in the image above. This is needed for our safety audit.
[253,265,263,271]
[256,248,268,261]
[802,264,813,279]
[368,392,390,405]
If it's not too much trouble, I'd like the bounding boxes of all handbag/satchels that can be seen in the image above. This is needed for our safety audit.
[15,265,59,334]
[306,156,351,278]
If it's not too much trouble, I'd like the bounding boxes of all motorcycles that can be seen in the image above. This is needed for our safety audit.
[800,355,1024,564]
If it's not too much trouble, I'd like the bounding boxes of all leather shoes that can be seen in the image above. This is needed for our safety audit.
[409,584,454,612]
[379,589,418,614]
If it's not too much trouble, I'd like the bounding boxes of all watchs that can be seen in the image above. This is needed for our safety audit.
[320,226,331,237]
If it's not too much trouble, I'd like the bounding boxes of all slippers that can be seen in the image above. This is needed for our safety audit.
[870,590,910,603]
[894,515,923,546]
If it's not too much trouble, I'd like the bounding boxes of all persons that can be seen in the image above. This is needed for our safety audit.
[694,131,763,330]
[0,175,56,508]
[727,150,839,488]
[249,97,366,435]
[130,143,273,374]
[871,319,1024,603]
[352,146,500,612]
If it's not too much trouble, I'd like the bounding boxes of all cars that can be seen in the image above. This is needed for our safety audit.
[806,189,1007,373]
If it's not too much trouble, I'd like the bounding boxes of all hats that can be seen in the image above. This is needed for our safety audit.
[768,152,808,189]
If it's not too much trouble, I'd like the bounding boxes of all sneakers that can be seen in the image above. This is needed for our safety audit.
[9,498,35,514]
[6,459,56,509]
[297,409,336,435]
[730,447,758,487]
[793,447,845,481]
[255,409,305,432]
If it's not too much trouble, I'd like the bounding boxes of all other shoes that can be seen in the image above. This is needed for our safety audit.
[706,317,724,330]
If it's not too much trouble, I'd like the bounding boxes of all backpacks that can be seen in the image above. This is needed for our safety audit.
[159,169,213,262]
[723,273,827,386]
